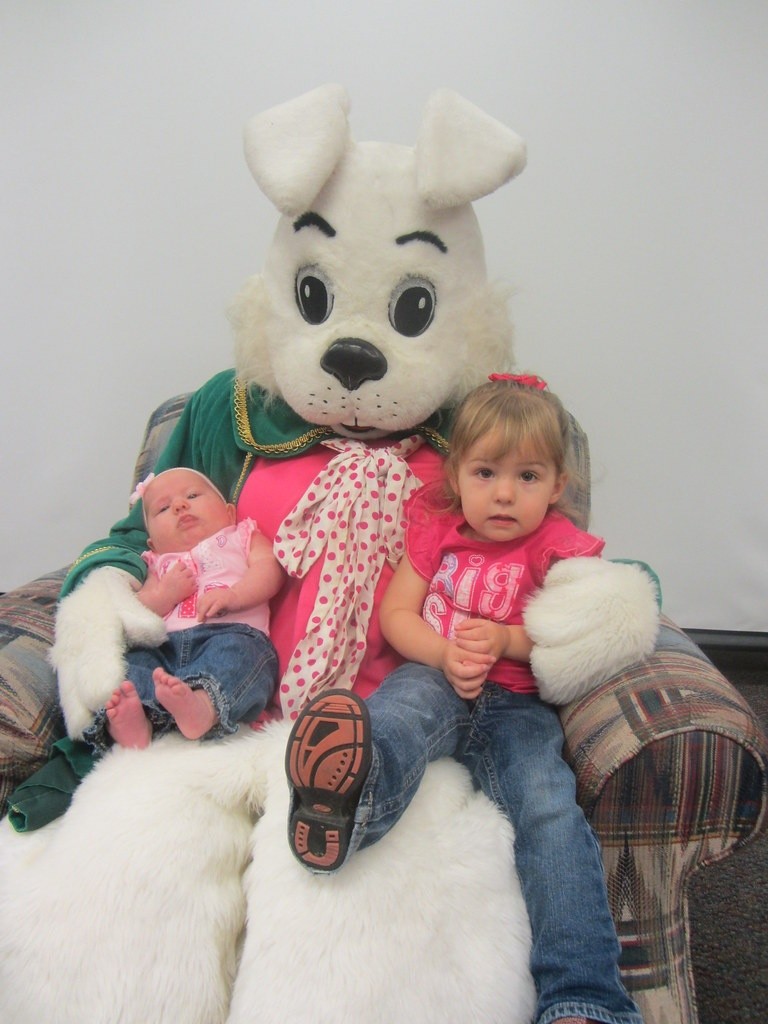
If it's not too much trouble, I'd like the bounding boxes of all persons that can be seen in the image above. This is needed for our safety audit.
[284,370,643,1024]
[110,461,282,738]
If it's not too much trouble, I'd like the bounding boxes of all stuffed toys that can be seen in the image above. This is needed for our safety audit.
[0,87,666,1023]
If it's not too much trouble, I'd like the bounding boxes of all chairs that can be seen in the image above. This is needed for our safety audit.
[5,388,768,1024]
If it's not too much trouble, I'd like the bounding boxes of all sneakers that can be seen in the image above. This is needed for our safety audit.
[284,688,372,872]
